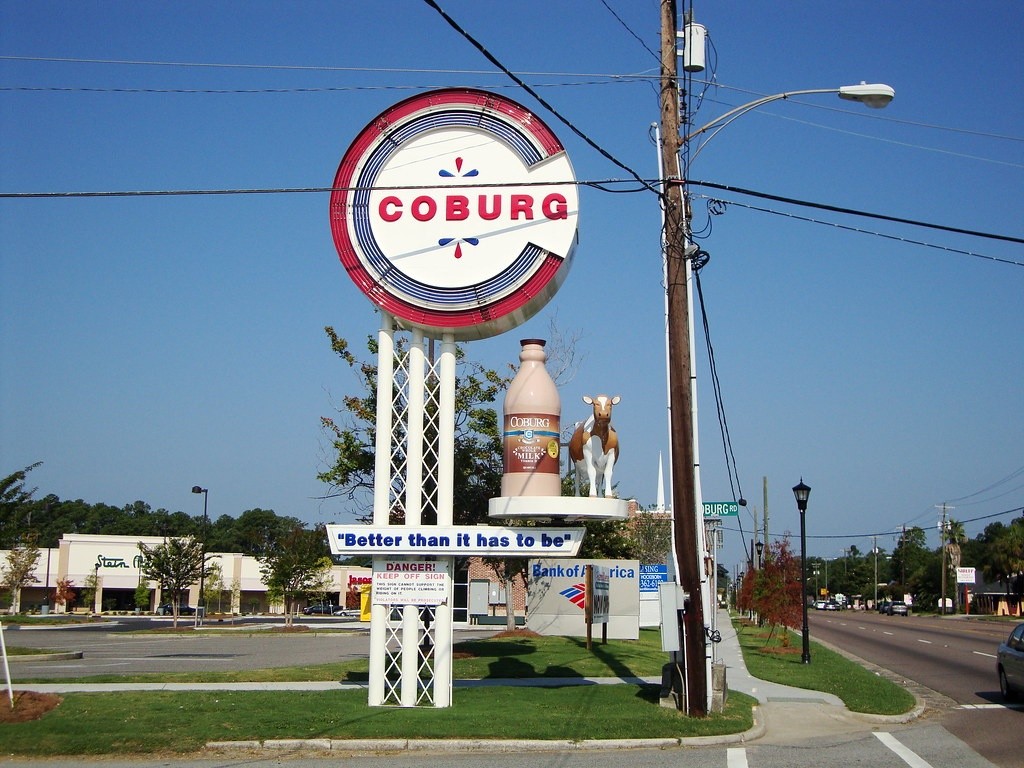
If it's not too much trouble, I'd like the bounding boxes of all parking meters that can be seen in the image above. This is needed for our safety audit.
[740,571,744,614]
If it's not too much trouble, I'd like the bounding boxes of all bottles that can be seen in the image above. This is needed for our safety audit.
[501,336,561,497]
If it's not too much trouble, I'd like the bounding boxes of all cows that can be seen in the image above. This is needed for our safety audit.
[569,395,623,498]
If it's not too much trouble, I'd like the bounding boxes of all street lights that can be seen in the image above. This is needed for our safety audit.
[92,563,100,613]
[793,478,813,667]
[659,79,898,721]
[191,485,209,618]
[754,539,765,628]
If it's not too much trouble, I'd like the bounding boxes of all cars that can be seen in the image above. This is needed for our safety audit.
[886,601,909,616]
[994,622,1024,700]
[301,605,333,615]
[333,606,361,616]
[815,600,840,611]
[162,602,196,616]
[879,601,891,615]
